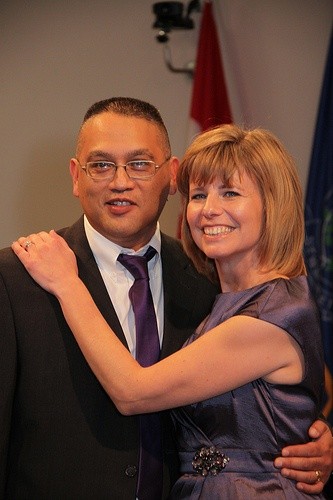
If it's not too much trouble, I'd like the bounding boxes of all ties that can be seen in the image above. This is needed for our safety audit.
[116,246,166,498]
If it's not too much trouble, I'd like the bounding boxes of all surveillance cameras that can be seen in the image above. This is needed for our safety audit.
[152,2,183,29]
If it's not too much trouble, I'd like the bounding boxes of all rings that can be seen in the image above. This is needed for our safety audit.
[24,242,36,251]
[315,470,321,484]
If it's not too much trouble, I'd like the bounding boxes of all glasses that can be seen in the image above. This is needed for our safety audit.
[75,155,170,179]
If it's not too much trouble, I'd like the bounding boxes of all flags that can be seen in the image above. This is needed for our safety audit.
[178,1,242,151]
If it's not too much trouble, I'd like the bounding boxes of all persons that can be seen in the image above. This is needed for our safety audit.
[1,96,333,500]
[11,123,327,500]
